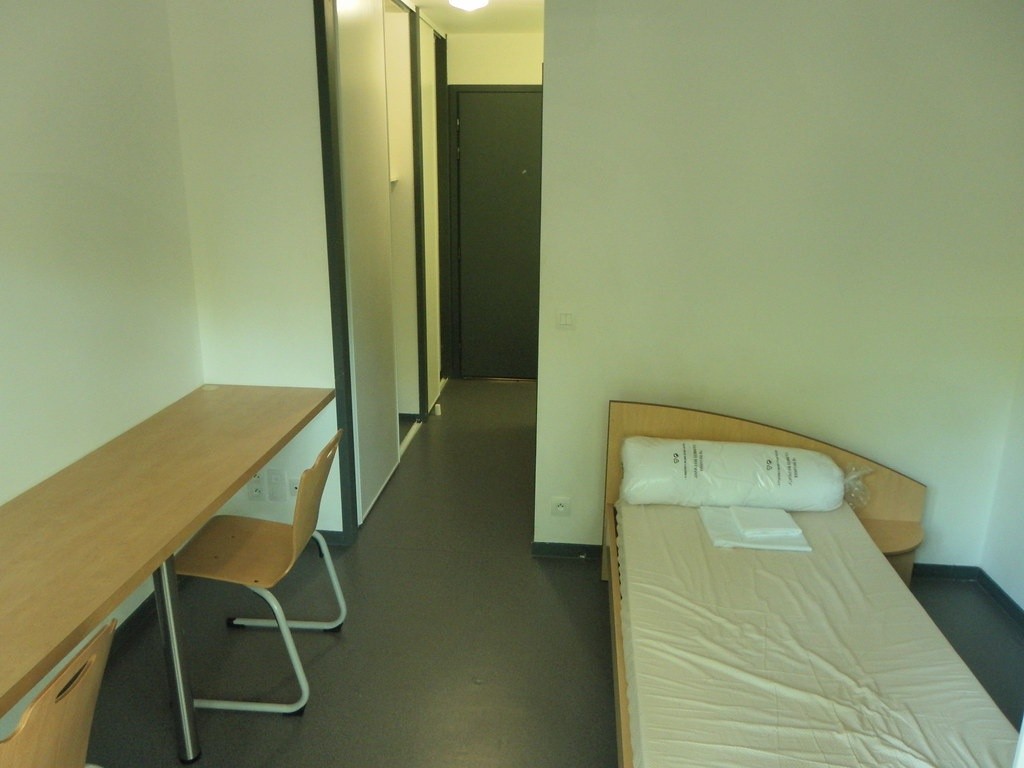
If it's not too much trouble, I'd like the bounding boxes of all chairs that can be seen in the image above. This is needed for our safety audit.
[1,618,117,767]
[172,428,347,716]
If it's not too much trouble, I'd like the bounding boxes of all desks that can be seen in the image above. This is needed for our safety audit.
[0,385,336,719]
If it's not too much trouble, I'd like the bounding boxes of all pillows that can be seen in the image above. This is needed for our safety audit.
[621,436,845,513]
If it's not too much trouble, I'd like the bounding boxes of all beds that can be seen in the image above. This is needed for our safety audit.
[603,399,1019,768]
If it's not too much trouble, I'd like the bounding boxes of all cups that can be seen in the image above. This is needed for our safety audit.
[435,403,441,415]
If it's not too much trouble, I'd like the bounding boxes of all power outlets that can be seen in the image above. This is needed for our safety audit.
[552,498,570,517]
